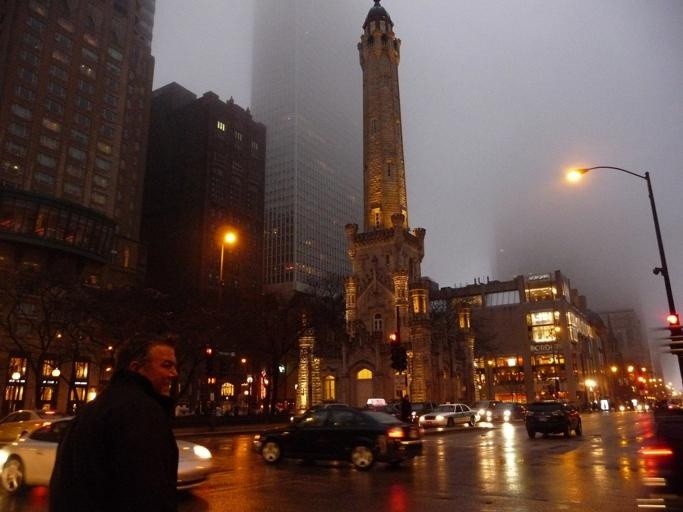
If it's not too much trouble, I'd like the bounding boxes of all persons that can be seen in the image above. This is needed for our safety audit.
[43,330,211,512]
[401,394,413,423]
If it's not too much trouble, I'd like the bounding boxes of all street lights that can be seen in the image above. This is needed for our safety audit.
[217,230,237,290]
[566,166,683,383]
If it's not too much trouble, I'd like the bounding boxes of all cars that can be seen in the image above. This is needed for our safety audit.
[525,400,583,437]
[1,409,214,489]
[253,403,423,471]
[410,400,528,430]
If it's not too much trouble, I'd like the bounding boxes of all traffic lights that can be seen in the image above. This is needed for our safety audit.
[390,333,399,343]
[667,314,679,325]
[390,347,408,370]
